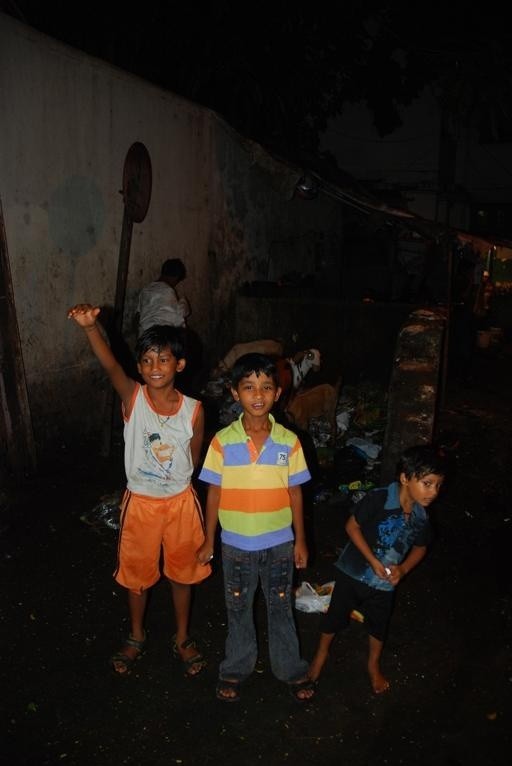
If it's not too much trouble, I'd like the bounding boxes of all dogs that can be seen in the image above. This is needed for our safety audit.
[279,375,343,448]
[200,336,283,401]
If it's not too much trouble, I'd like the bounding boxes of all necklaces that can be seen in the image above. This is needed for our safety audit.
[148,391,175,426]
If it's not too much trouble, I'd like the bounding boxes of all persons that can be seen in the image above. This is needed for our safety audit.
[132,258,188,378]
[68,303,211,677]
[308,446,445,694]
[196,352,315,703]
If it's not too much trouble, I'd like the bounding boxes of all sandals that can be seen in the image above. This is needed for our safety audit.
[170,636,207,678]
[287,672,320,703]
[111,628,147,673]
[216,674,240,702]
